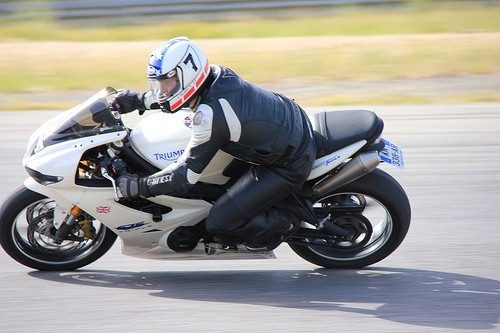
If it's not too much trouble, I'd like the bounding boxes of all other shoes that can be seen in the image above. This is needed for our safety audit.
[245,212,301,252]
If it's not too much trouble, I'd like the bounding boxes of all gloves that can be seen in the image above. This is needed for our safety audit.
[117,173,156,200]
[112,90,149,116]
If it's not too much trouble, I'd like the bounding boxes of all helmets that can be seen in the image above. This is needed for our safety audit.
[148,35,213,115]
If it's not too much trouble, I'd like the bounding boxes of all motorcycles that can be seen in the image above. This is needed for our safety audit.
[0,86,411,271]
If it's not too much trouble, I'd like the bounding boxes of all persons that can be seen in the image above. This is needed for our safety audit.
[110,37,317,252]
